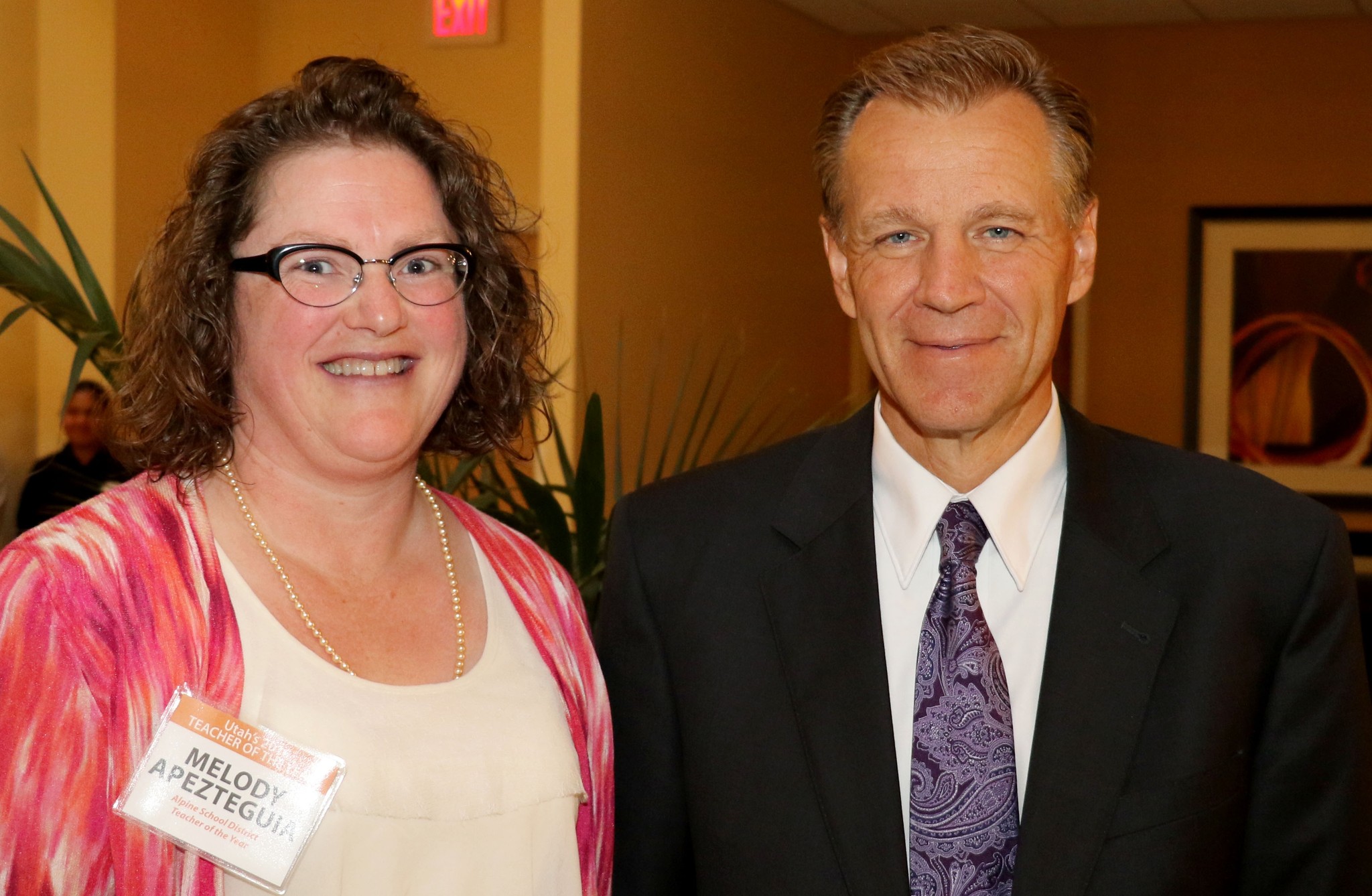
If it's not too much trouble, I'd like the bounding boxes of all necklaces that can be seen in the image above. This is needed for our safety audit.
[216,444,465,683]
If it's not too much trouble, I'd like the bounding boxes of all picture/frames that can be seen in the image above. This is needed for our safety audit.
[852,294,1091,422]
[1182,204,1372,514]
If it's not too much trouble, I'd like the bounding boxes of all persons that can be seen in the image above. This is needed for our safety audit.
[0,52,616,896]
[597,25,1372,895]
[13,381,130,534]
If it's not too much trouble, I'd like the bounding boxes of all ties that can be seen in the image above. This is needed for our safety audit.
[909,504,1019,896]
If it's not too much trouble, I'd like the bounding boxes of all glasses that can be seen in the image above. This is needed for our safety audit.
[234,242,476,308]
